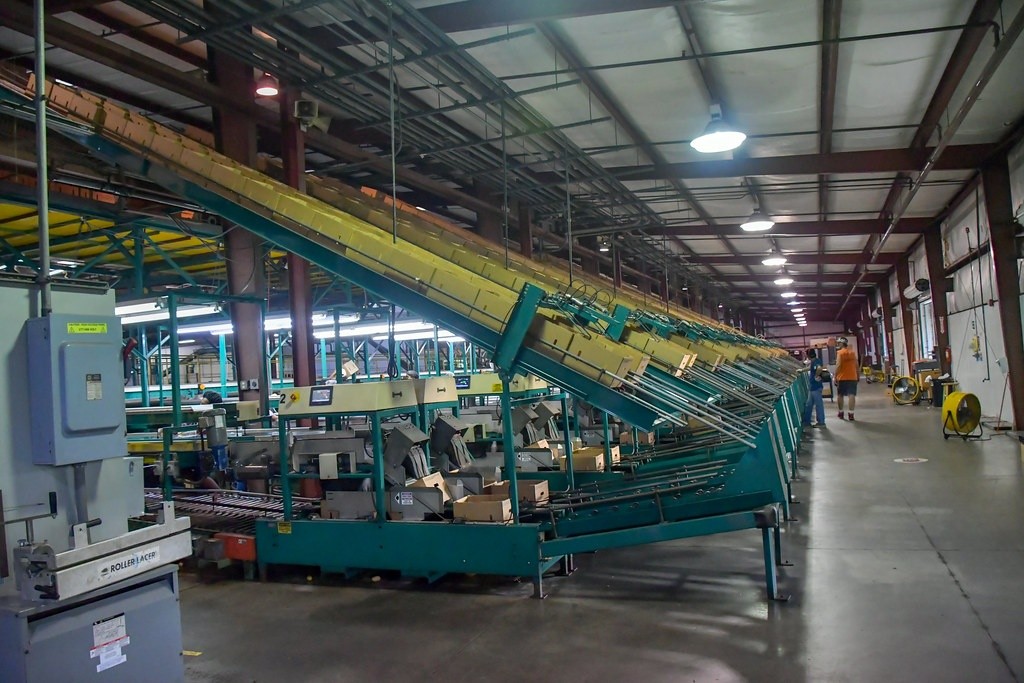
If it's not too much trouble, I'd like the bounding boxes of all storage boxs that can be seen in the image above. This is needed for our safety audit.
[321,410,722,525]
[331,360,359,381]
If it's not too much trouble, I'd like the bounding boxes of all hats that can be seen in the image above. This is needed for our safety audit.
[836,337,848,343]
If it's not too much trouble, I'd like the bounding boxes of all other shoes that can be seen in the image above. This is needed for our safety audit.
[848,413,854,420]
[838,411,844,418]
[813,422,825,427]
[802,426,811,432]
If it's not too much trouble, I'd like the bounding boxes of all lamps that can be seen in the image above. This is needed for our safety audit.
[741,206,808,340]
[254,64,279,97]
[690,104,747,156]
[115,295,467,344]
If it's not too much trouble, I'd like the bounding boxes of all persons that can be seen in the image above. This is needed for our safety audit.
[833,337,860,420]
[200,391,222,406]
[796,349,826,427]
[406,370,418,379]
